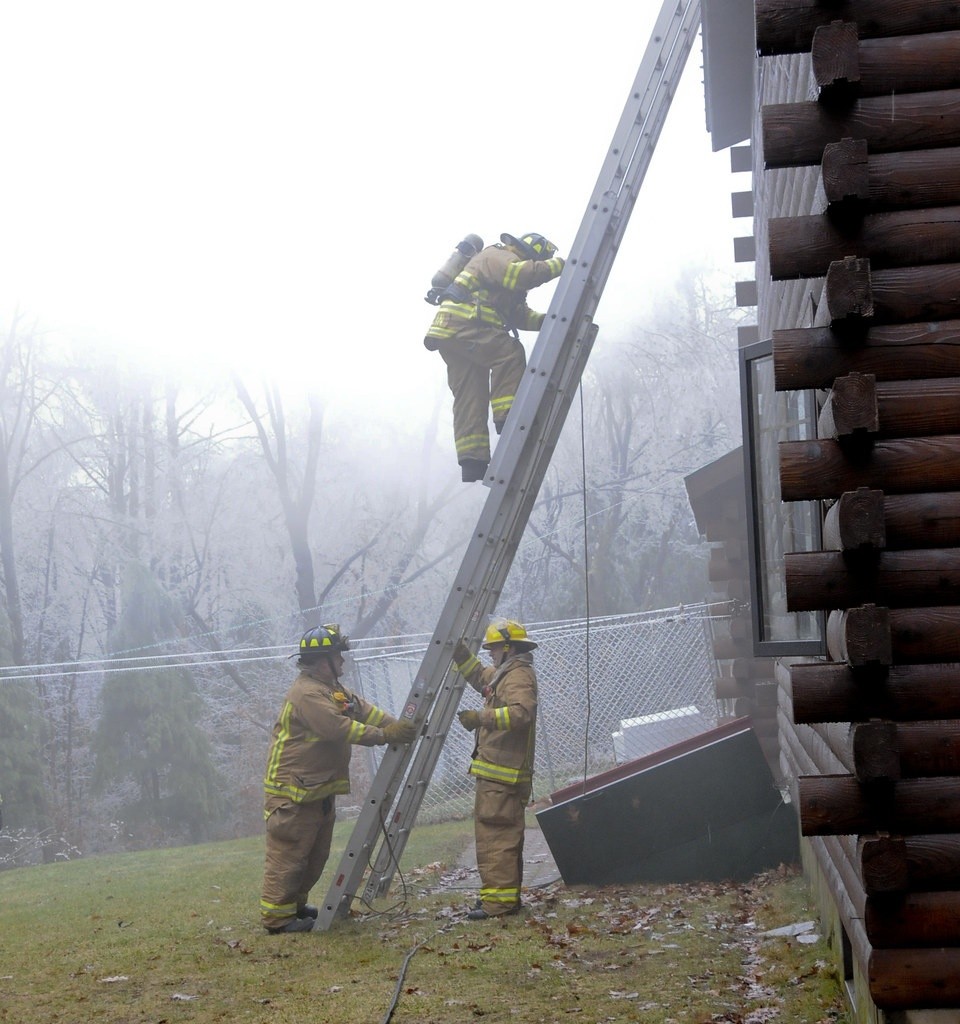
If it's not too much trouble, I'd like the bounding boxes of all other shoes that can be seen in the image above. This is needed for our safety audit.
[475,897,483,909]
[462,461,488,482]
[495,422,504,434]
[468,909,491,919]
[296,904,318,920]
[269,918,315,934]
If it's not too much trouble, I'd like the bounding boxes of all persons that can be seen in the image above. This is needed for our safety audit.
[257,623,415,933]
[425,231,568,483]
[454,620,541,918]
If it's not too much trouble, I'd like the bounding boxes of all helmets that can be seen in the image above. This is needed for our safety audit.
[482,614,538,651]
[288,623,351,660]
[500,232,559,262]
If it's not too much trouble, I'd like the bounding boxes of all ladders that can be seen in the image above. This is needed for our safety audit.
[311,0,707,937]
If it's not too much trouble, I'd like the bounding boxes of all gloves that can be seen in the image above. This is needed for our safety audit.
[457,710,478,732]
[383,721,416,743]
[420,719,429,736]
[453,642,470,666]
